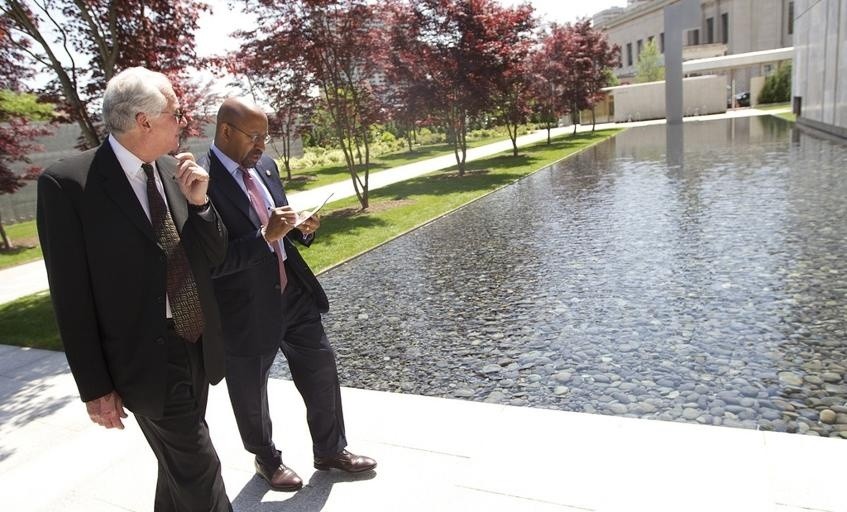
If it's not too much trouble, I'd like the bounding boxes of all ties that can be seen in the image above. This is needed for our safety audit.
[237,165,288,295]
[142,163,207,344]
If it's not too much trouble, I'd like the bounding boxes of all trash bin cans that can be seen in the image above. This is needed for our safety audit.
[793,97,801,115]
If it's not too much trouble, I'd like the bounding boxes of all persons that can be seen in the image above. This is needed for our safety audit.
[192,96,377,492]
[34,66,233,510]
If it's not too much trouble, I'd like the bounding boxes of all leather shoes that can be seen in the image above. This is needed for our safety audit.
[314,448,378,475]
[254,458,302,493]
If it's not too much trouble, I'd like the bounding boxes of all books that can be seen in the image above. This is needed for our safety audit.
[275,191,333,235]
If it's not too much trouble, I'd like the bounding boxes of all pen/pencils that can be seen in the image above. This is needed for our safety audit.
[268,207,273,211]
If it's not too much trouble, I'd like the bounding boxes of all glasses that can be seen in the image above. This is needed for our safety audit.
[227,123,271,145]
[160,105,183,124]
